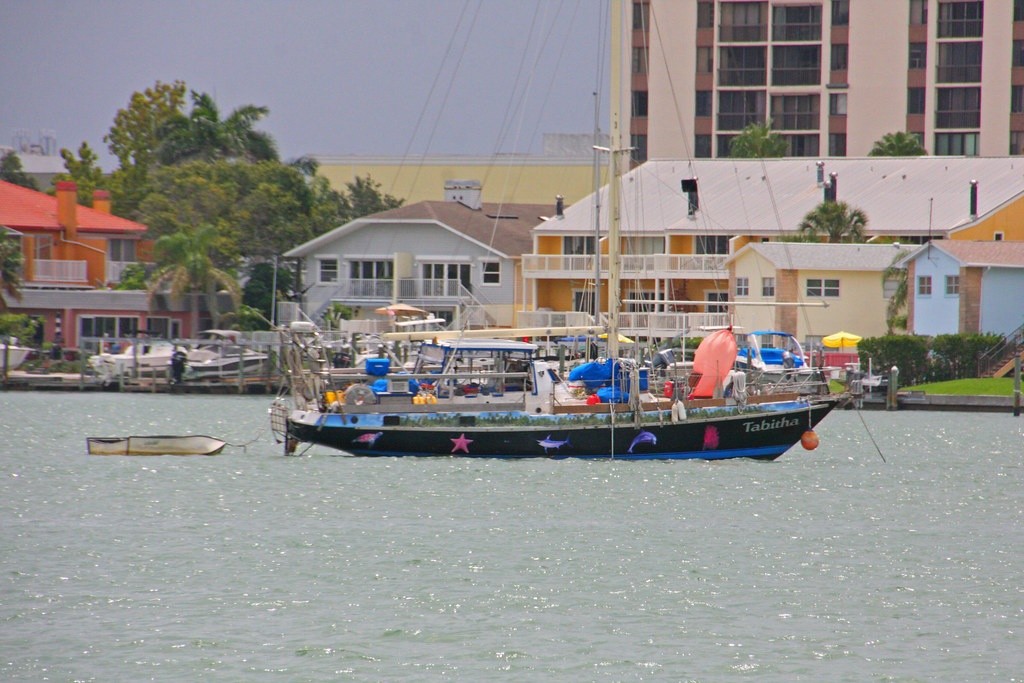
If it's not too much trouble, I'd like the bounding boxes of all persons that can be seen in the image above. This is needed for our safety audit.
[783,351,797,382]
[170,346,186,381]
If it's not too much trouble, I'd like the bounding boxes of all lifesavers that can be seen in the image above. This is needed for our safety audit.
[344,383,376,414]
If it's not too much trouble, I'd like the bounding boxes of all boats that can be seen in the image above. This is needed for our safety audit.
[526,328,651,392]
[734,330,881,395]
[85,436,227,456]
[88,335,270,375]
[0,343,35,375]
[361,303,539,366]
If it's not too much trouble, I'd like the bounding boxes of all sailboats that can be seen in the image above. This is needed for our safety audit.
[270,1,895,464]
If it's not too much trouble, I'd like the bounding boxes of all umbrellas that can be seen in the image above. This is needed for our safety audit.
[375,303,427,316]
[597,331,635,343]
[821,331,862,353]
